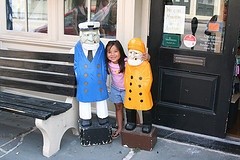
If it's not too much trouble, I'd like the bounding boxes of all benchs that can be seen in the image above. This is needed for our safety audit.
[0,49,79,158]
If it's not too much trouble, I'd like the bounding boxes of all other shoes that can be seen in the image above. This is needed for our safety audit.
[112,131,121,139]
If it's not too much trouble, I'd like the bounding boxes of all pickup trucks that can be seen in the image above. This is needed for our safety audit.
[34,6,116,37]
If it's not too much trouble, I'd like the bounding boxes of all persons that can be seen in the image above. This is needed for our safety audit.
[105,40,151,139]
[205,15,218,51]
[93,0,111,38]
[97,29,107,38]
[73,22,110,127]
[73,0,88,36]
[125,37,153,133]
